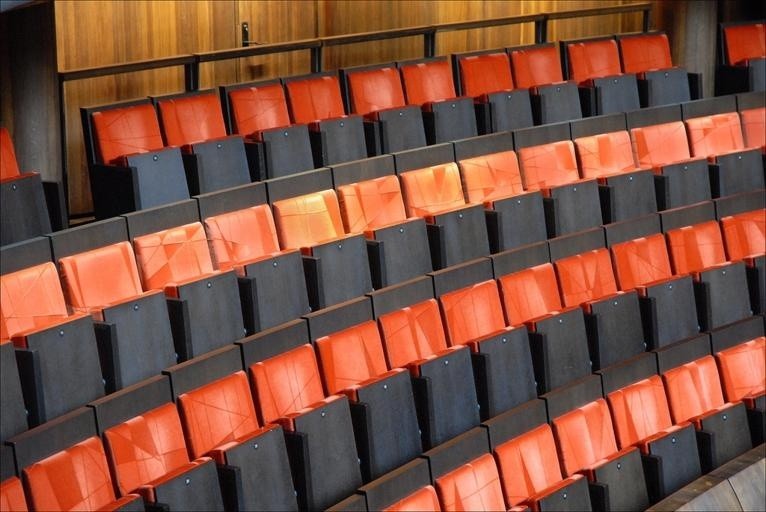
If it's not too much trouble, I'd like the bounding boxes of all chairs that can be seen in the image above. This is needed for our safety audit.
[0,22,766,511]
